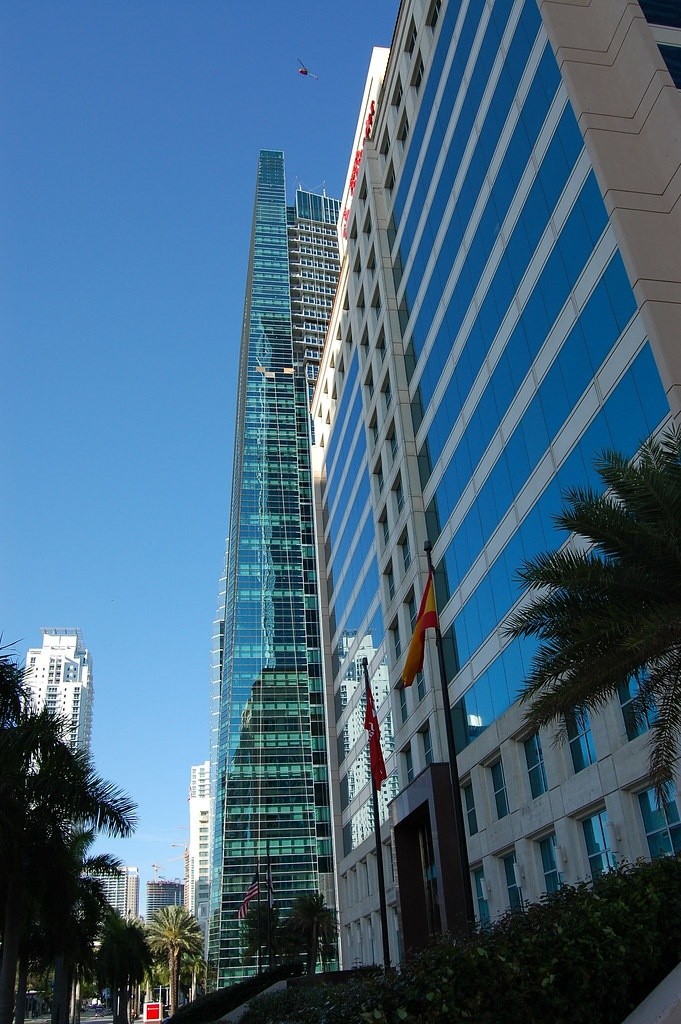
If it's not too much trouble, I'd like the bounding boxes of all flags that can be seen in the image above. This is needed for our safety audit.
[394,569,439,689]
[364,673,388,791]
[238,863,274,919]
